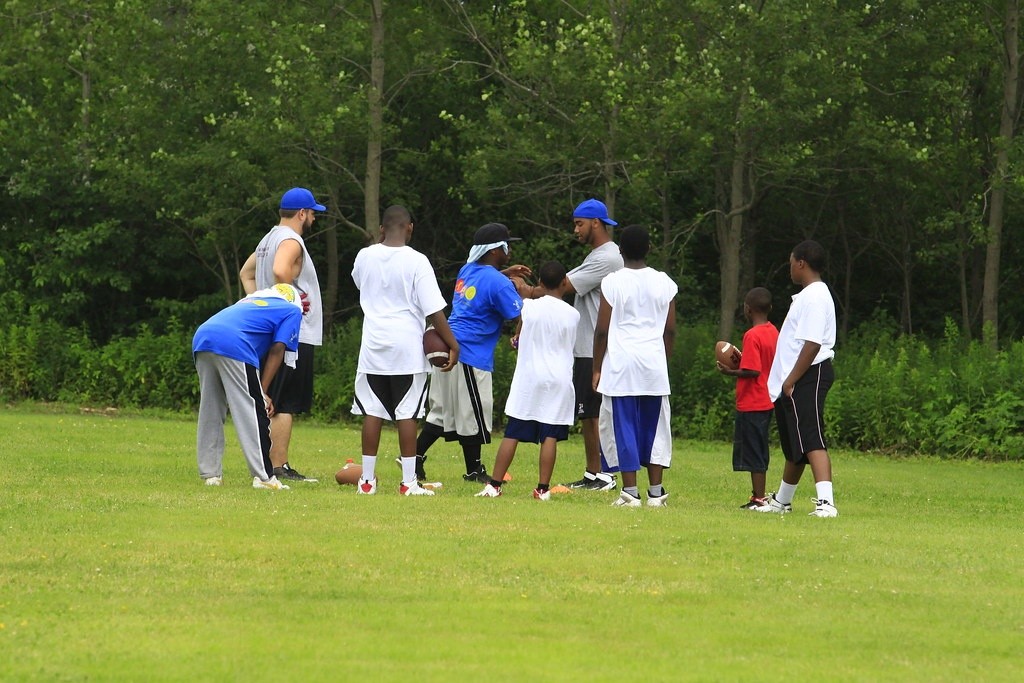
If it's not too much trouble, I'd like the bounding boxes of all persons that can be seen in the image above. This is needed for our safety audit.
[510,198,624,491]
[592,225,678,507]
[351,204,460,496]
[753,239,837,517]
[395,221,533,487]
[191,283,303,490]
[717,288,780,510]
[474,261,581,501]
[240,188,326,483]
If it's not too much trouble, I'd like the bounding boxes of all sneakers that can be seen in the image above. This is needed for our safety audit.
[557,467,596,490]
[474,482,502,498]
[273,462,320,483]
[610,486,642,507]
[808,497,839,517]
[645,486,669,507]
[533,488,551,501]
[252,473,291,490]
[755,490,793,514]
[356,473,376,495]
[586,472,618,491]
[740,491,772,511]
[462,464,492,482]
[395,454,428,482]
[205,474,224,486]
[399,480,435,496]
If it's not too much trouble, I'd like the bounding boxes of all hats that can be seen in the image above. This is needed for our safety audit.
[280,187,326,211]
[474,222,522,245]
[573,198,618,226]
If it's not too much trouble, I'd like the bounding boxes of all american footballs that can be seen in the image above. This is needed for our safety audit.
[716,340,741,371]
[335,464,378,486]
[422,321,450,368]
